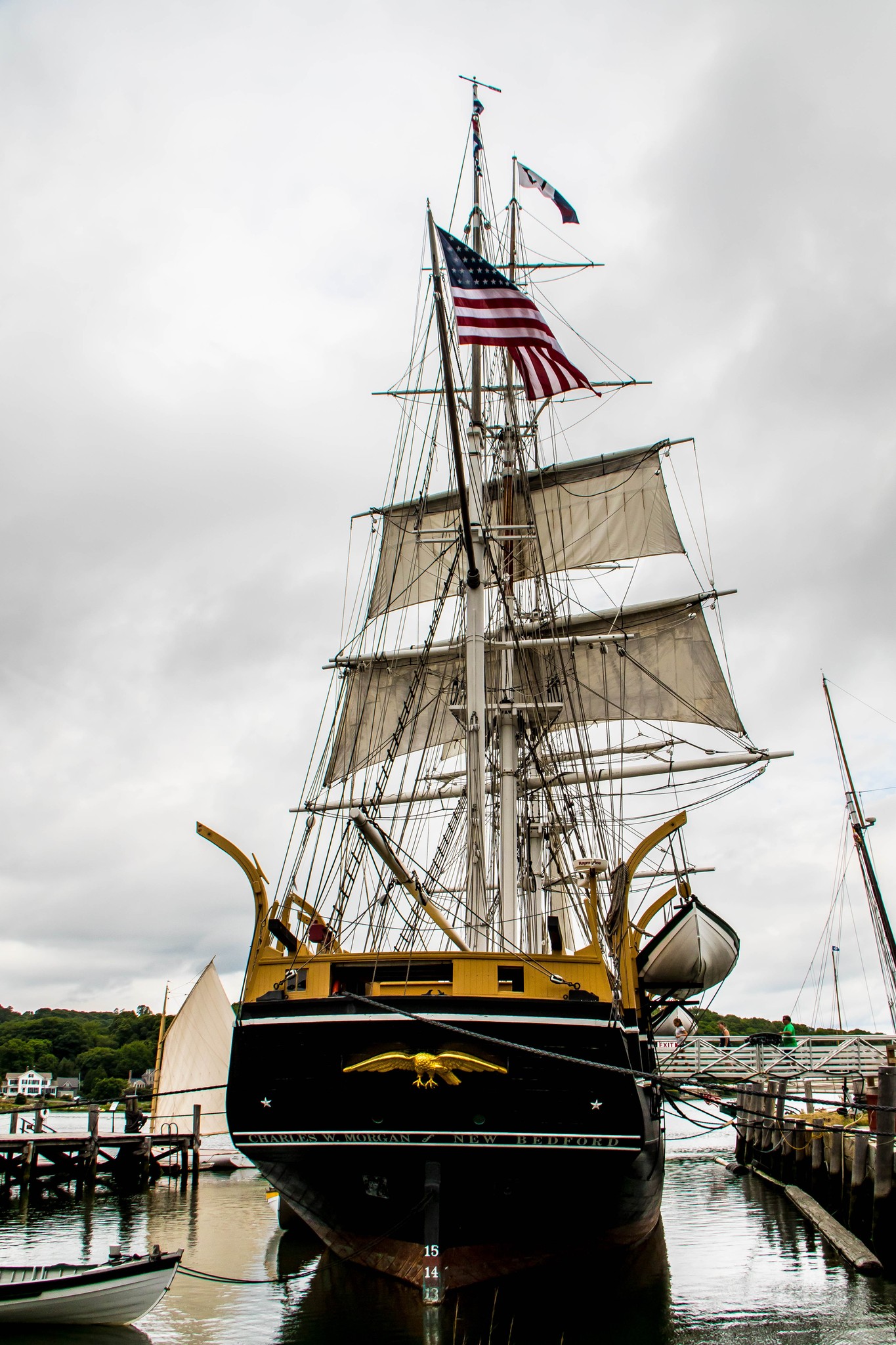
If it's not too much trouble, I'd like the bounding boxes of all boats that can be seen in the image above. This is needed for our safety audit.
[0,1243,185,1326]
[637,897,740,1001]
[649,1005,699,1038]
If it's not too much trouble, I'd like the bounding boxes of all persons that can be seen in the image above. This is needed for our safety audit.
[777,1016,798,1073]
[717,1021,737,1073]
[672,1018,692,1070]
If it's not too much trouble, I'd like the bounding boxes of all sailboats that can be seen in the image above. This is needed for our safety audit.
[189,77,795,1309]
[143,955,259,1170]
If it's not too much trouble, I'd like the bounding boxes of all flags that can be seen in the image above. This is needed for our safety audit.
[473,91,484,177]
[699,1093,722,1107]
[434,221,602,399]
[517,161,580,225]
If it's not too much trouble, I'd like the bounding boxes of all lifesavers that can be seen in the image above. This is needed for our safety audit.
[41,1108,50,1118]
